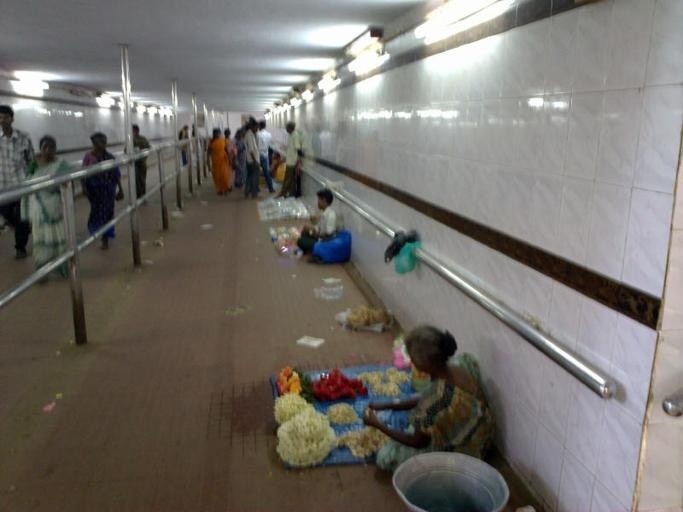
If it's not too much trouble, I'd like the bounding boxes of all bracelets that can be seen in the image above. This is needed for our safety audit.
[392,399,401,412]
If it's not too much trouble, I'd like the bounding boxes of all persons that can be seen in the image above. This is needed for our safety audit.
[297,188,337,255]
[0,103,35,259]
[20,133,75,280]
[363,324,497,472]
[80,132,125,250]
[132,123,150,199]
[207,119,276,198]
[178,124,189,167]
[274,120,303,199]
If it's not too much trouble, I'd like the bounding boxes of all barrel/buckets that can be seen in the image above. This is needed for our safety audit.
[392,450,510,512]
